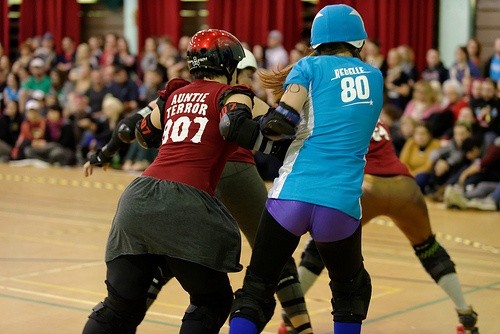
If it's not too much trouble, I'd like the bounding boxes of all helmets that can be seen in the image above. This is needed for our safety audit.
[310,4,367,49]
[186,29,244,77]
[235,47,258,71]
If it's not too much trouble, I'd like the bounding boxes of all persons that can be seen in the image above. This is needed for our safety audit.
[0,26,500,212]
[278,52,478,334]
[84,47,314,334]
[229,4,383,334]
[81,28,296,334]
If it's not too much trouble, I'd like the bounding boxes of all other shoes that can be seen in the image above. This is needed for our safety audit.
[421,175,497,212]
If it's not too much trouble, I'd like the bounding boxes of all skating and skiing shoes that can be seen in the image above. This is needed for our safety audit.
[277,310,294,334]
[455,305,478,334]
